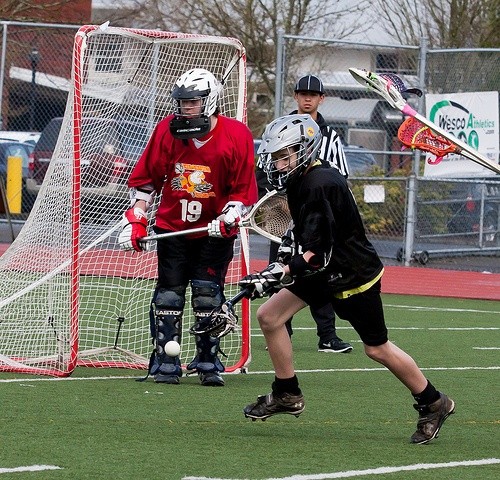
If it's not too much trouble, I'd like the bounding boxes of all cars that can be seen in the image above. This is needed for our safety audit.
[0,130,42,215]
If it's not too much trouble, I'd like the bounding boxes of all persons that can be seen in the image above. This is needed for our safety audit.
[254,75,354,353]
[244,113,454,445]
[117,69,258,386]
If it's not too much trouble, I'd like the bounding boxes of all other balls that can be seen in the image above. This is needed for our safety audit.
[165,341,181,356]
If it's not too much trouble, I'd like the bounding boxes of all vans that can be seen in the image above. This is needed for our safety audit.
[27,117,160,209]
[253,137,378,207]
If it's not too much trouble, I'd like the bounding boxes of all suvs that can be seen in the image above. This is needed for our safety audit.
[447,180,500,246]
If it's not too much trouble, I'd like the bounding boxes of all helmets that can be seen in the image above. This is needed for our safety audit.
[170,68,220,139]
[257,114,323,190]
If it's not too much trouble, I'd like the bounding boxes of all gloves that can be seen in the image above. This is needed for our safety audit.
[208,201,247,239]
[119,207,149,252]
[238,262,295,301]
[275,229,298,265]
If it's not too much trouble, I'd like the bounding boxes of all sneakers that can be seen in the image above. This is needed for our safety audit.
[154,371,180,384]
[410,390,456,445]
[318,338,353,353]
[264,335,292,350]
[243,391,305,421]
[198,370,225,384]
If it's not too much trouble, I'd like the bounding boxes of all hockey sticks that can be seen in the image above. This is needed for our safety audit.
[192,283,249,341]
[137,187,295,244]
[395,115,500,174]
[349,66,500,173]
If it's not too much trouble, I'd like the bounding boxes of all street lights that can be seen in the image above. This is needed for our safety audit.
[28,46,41,129]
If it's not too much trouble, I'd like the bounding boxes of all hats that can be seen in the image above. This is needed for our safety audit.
[294,74,324,94]
